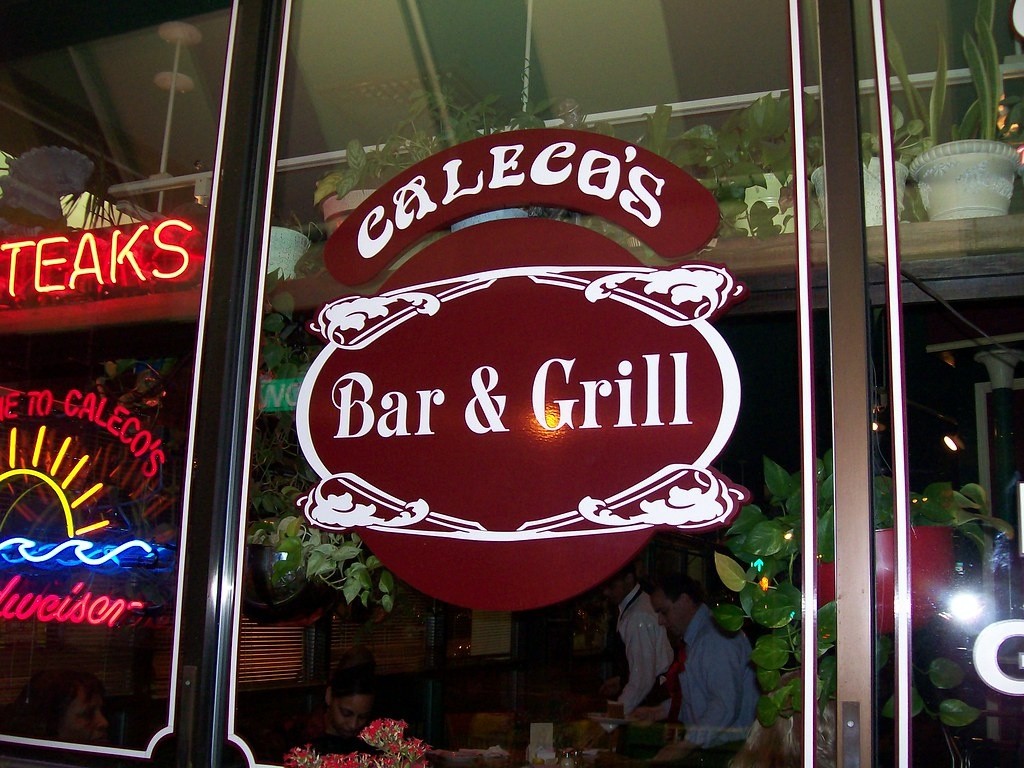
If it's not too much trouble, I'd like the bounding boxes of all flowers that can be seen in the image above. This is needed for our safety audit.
[282,717,432,768]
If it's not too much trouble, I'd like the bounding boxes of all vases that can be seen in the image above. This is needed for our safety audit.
[266,225,311,283]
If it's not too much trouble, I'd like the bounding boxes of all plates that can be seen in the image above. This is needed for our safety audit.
[582,711,637,726]
[436,749,480,761]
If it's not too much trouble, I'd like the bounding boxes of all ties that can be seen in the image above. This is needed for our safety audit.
[664,645,687,723]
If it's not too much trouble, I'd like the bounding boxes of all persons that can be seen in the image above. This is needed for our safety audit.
[729,669,837,768]
[576,566,679,760]
[626,572,760,768]
[0,662,109,768]
[279,644,384,757]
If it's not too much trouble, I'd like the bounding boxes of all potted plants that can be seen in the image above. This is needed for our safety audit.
[709,444,1019,768]
[246,290,402,613]
[313,0,1024,246]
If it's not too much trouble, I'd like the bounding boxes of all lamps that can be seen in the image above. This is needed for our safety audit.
[869,421,887,433]
[931,435,960,458]
[925,351,956,371]
